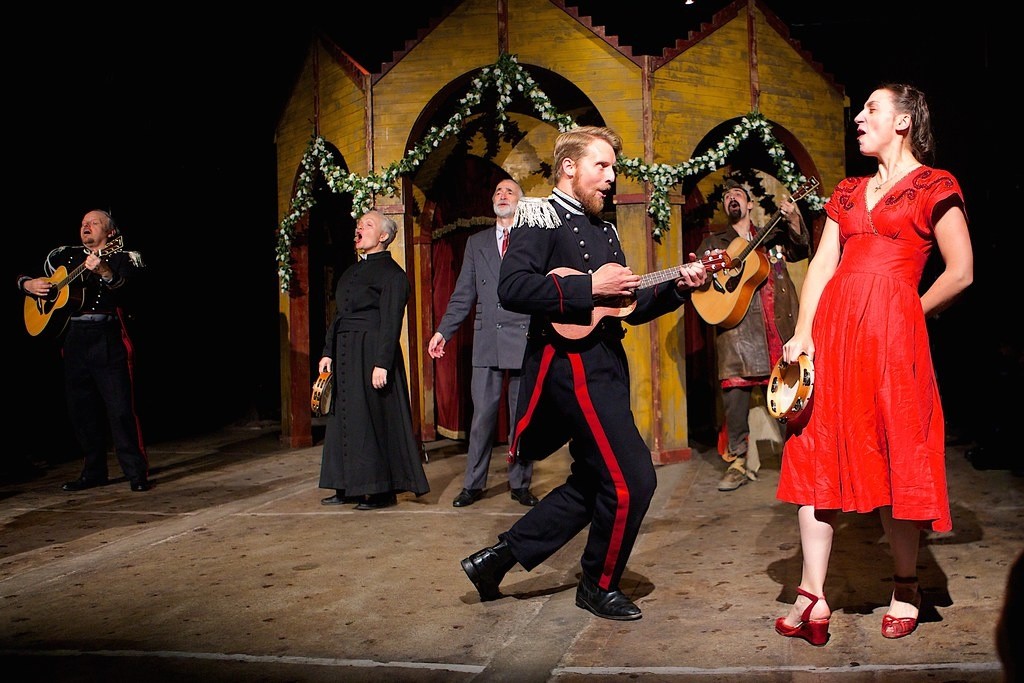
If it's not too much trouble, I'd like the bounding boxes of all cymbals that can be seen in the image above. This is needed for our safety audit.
[311,372,334,418]
[766,354,815,425]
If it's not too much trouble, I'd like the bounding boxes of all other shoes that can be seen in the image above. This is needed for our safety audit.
[718,465,748,490]
[320,495,366,505]
[356,498,397,510]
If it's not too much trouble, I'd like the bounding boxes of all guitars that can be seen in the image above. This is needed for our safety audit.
[544,248,733,352]
[690,175,821,329]
[23,235,124,339]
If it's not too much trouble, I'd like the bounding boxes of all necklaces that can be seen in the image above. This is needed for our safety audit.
[874,163,919,193]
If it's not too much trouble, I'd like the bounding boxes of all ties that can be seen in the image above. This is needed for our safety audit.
[501,230,510,259]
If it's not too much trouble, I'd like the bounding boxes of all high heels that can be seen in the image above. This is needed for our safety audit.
[882,582,923,638]
[775,585,832,645]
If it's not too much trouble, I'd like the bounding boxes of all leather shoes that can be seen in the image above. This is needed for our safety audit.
[511,487,539,506]
[576,574,642,619]
[452,488,483,507]
[461,547,505,602]
[62,477,87,490]
[130,476,147,491]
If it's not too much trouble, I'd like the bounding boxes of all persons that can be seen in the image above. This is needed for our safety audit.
[459,125,706,615]
[693,185,800,491]
[773,86,973,643]
[17,210,151,491]
[428,178,541,504]
[318,210,431,509]
